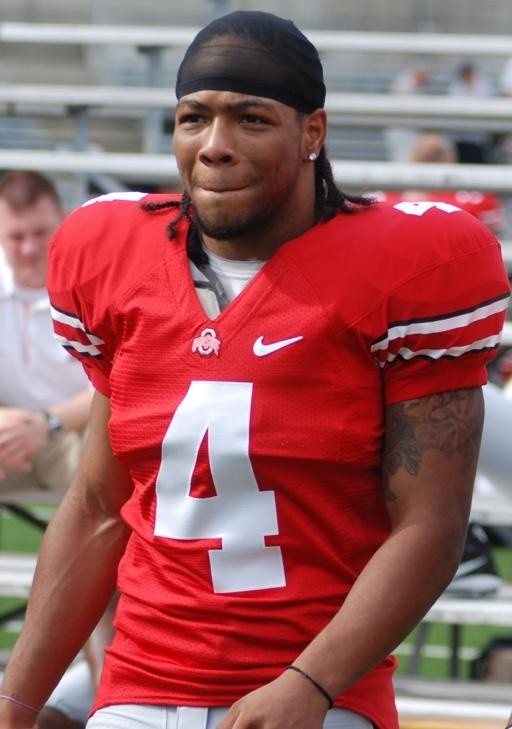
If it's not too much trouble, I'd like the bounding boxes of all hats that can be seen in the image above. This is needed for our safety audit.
[176,11,325,113]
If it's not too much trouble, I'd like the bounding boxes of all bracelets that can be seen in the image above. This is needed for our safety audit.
[281,661,335,710]
[1,694,43,717]
[39,408,68,448]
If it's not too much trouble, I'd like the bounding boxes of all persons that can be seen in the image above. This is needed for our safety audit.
[370,39,511,166]
[1,8,511,729]
[2,167,104,495]
